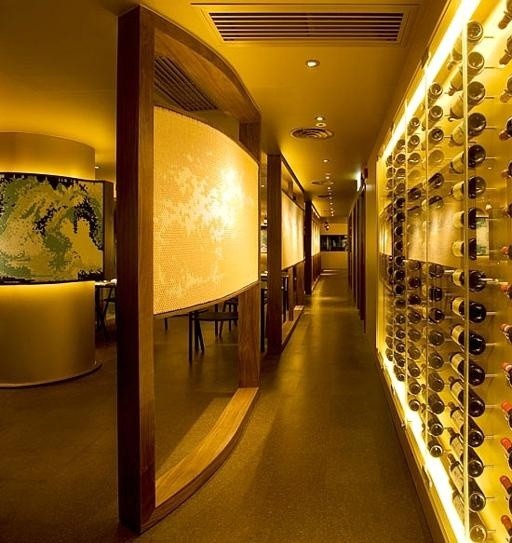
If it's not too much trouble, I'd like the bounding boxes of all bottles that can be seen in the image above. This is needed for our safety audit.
[494,1,512,543]
[379,17,486,543]
